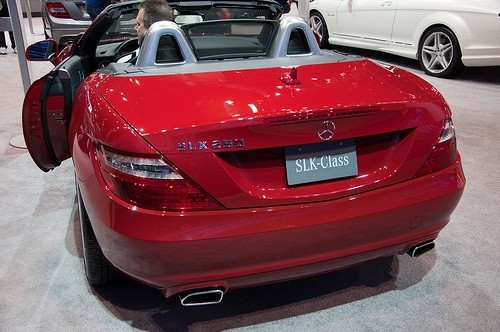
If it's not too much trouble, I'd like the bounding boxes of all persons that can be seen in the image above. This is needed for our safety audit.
[116,0,176,63]
[0,0,18,55]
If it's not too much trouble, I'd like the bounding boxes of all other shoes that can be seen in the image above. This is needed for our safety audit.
[0,47,7,53]
[13,47,17,54]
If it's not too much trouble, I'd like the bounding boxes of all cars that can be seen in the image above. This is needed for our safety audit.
[41,0,92,44]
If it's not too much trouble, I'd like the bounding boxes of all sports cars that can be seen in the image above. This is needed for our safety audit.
[22,0,466,306]
[309,0,500,77]
